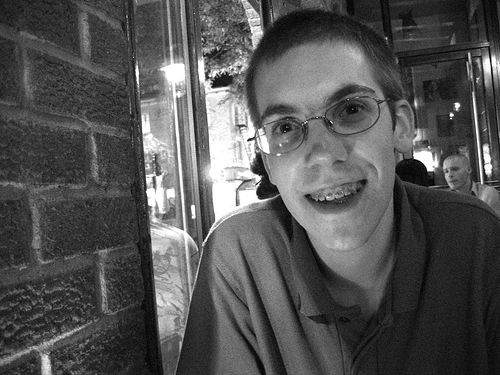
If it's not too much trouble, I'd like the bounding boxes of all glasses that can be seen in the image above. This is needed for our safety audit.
[247,96,391,155]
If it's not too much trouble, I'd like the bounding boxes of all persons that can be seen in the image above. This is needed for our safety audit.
[441,155,500,217]
[175,7,500,375]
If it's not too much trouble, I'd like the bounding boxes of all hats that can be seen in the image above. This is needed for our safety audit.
[395,158,435,186]
